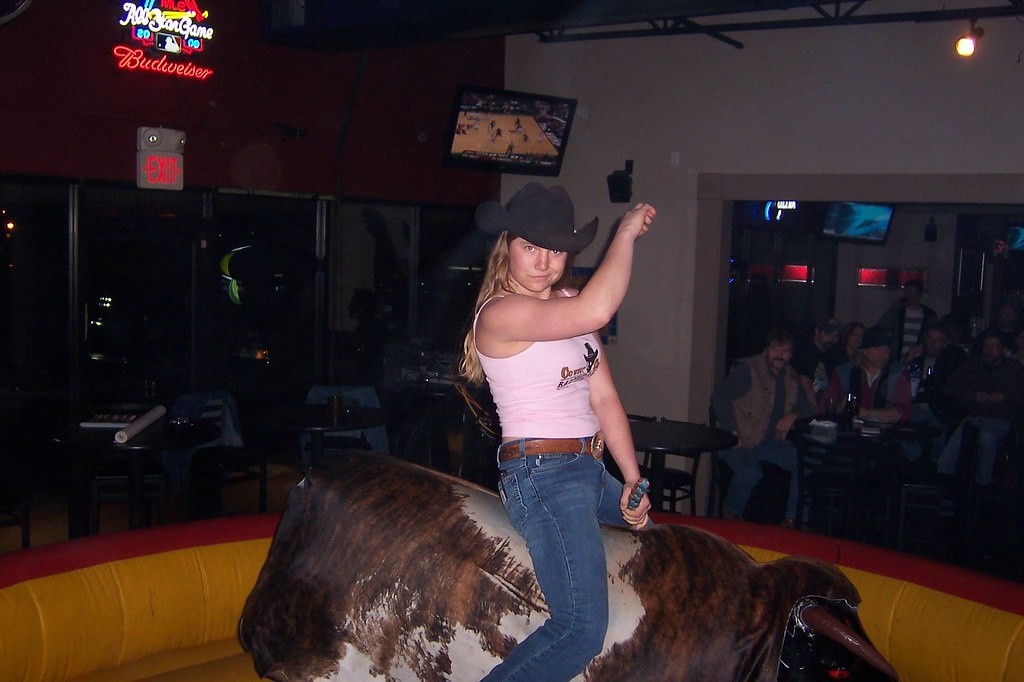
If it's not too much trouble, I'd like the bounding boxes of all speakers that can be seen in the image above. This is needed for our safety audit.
[608,169,632,202]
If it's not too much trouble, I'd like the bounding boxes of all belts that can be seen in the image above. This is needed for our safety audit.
[499,431,604,462]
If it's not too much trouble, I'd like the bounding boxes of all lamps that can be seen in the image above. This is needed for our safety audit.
[955,22,985,57]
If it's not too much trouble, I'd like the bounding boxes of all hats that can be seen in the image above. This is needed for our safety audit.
[813,316,844,332]
[858,324,894,349]
[474,182,599,253]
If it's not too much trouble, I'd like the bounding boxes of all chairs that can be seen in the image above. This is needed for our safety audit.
[642,417,707,516]
[627,413,658,476]
[0,428,34,548]
[794,414,871,540]
[81,396,169,534]
[175,391,269,516]
[894,426,985,567]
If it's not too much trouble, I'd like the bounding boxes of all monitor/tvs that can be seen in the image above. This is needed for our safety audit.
[819,201,897,246]
[442,84,578,178]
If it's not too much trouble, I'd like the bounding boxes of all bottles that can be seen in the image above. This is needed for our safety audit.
[841,392,853,432]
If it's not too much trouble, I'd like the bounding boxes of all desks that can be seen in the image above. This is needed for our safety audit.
[629,421,739,518]
[793,417,941,547]
[46,419,223,535]
[254,406,407,458]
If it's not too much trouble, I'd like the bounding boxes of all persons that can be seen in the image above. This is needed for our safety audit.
[462,181,657,682]
[711,279,1023,524]
[450,90,572,165]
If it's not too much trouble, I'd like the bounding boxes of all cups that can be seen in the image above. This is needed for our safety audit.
[854,421,865,432]
[911,380,919,396]
